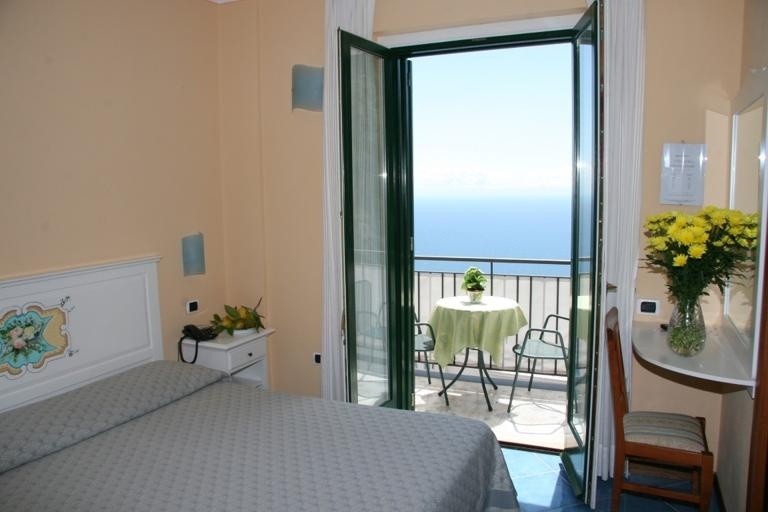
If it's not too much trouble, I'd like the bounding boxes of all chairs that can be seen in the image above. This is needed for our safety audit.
[506,313,579,414]
[354,280,386,363]
[379,300,450,407]
[605,306,713,512]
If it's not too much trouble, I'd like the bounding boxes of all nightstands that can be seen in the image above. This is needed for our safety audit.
[177,328,277,391]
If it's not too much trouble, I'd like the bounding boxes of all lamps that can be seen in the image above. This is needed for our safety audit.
[292,64,324,112]
[182,233,206,276]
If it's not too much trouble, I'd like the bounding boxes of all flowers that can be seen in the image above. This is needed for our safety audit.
[638,205,747,314]
[747,210,760,290]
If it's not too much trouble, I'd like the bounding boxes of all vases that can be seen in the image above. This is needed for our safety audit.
[666,301,708,356]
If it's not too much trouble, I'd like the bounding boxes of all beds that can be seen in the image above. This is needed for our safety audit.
[0,255,521,512]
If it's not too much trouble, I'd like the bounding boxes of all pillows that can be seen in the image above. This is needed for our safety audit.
[1,360,230,476]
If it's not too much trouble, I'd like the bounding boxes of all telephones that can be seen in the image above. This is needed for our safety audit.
[181,324,218,341]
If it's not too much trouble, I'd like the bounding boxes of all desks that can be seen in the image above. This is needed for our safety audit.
[631,322,753,394]
[425,295,528,410]
[577,293,591,344]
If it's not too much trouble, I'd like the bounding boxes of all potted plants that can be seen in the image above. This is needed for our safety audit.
[461,266,488,304]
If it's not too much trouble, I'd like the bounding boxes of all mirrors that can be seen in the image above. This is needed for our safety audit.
[723,91,766,350]
